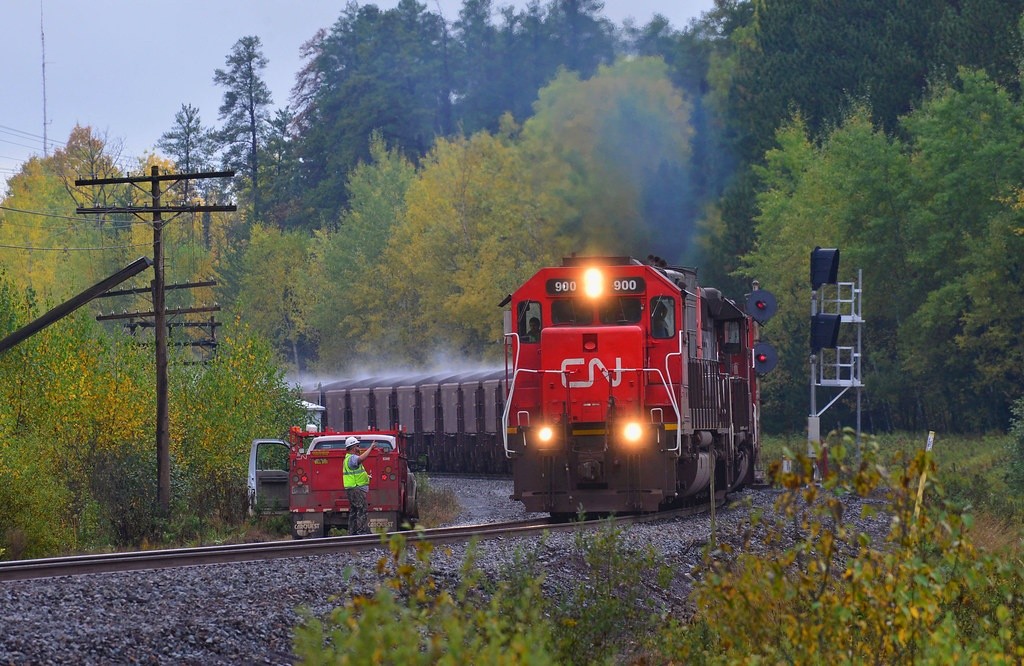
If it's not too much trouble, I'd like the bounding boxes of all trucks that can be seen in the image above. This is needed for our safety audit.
[247,425,419,539]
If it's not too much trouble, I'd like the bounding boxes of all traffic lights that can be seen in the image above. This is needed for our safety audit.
[754,344,778,374]
[813,247,840,284]
[746,289,777,322]
[815,313,841,349]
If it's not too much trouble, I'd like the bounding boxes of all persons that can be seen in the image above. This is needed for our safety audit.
[653,301,669,337]
[342,436,378,535]
[526,317,542,343]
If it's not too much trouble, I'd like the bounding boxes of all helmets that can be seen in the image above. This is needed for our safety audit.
[345,437,360,447]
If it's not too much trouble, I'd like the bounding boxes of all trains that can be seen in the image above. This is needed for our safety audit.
[288,251,755,522]
